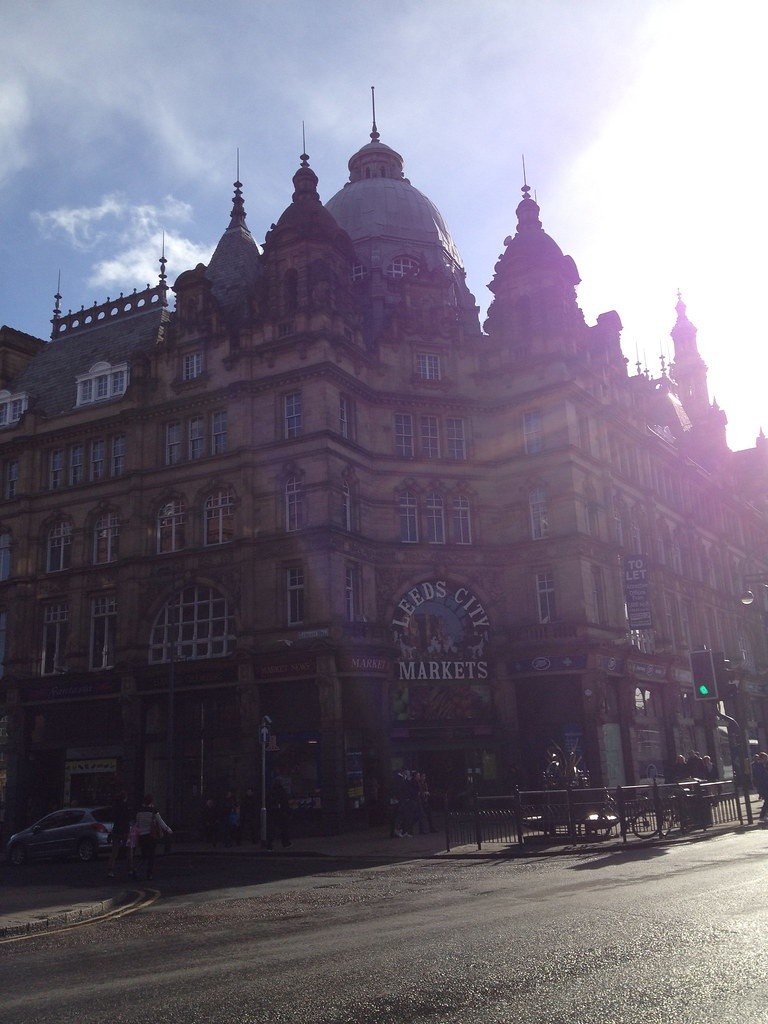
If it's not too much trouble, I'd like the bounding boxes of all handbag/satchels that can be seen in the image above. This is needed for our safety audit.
[149,807,164,839]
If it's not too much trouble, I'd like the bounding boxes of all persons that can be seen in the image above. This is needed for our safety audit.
[108,792,173,880]
[675,750,719,806]
[203,789,259,848]
[751,752,768,820]
[386,770,440,837]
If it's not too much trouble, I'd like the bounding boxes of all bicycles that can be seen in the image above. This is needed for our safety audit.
[578,785,663,841]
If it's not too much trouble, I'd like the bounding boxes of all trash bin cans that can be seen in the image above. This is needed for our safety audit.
[675,788,714,833]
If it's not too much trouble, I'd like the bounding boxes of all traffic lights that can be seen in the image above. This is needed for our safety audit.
[689,650,718,702]
[714,652,739,700]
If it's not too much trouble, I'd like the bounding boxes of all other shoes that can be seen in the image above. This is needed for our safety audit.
[108,873,115,878]
[400,832,413,838]
[128,871,134,876]
[419,829,427,834]
[430,828,439,832]
[286,842,293,848]
[267,848,275,852]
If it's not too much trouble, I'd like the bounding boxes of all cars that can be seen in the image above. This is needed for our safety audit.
[5,807,134,862]
